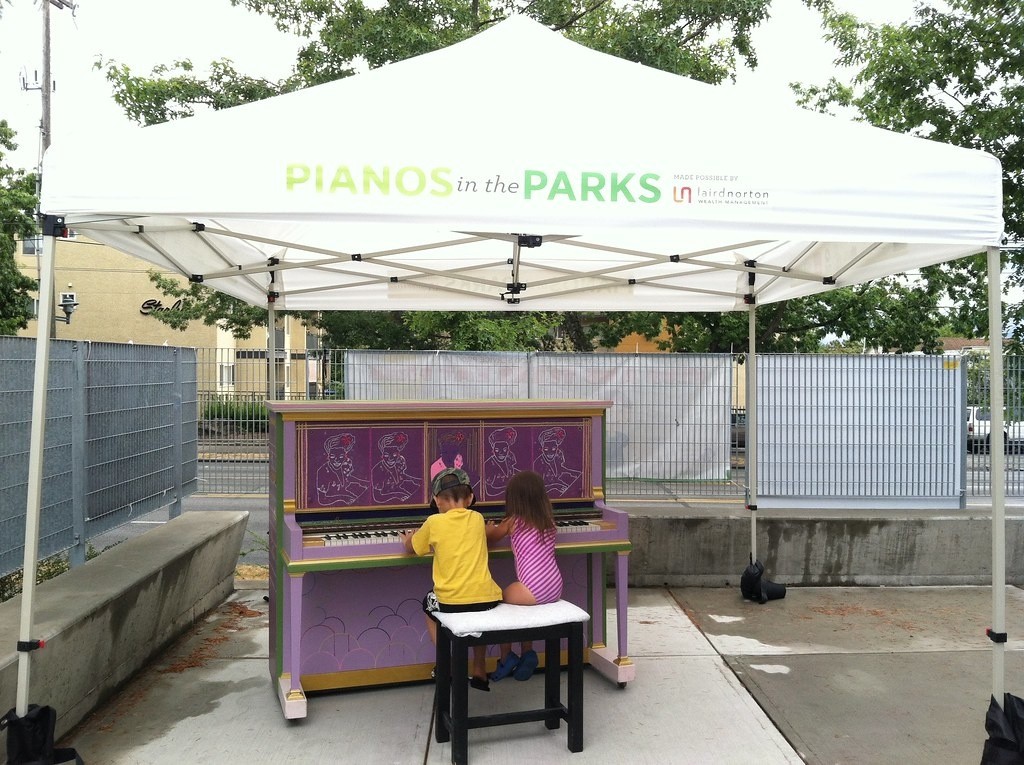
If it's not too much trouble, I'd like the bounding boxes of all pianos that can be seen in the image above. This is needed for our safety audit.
[263,398,634,720]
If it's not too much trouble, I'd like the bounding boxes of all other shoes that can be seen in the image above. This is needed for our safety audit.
[431,664,437,677]
[470,675,490,692]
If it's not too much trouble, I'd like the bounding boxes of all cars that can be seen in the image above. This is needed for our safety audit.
[731,413,746,449]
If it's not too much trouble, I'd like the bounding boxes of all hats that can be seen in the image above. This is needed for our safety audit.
[430,467,476,514]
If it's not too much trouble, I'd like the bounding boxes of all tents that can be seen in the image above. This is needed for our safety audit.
[15,13,1008,720]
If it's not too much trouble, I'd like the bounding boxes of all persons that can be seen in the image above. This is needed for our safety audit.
[486,471,563,683]
[400,468,503,692]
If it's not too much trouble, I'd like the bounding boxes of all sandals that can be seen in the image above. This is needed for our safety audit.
[490,652,520,682]
[514,650,538,681]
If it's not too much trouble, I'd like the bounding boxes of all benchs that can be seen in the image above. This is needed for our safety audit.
[423,601,591,765]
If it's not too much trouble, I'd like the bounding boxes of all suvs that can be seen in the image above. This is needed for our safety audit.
[965,405,1024,454]
